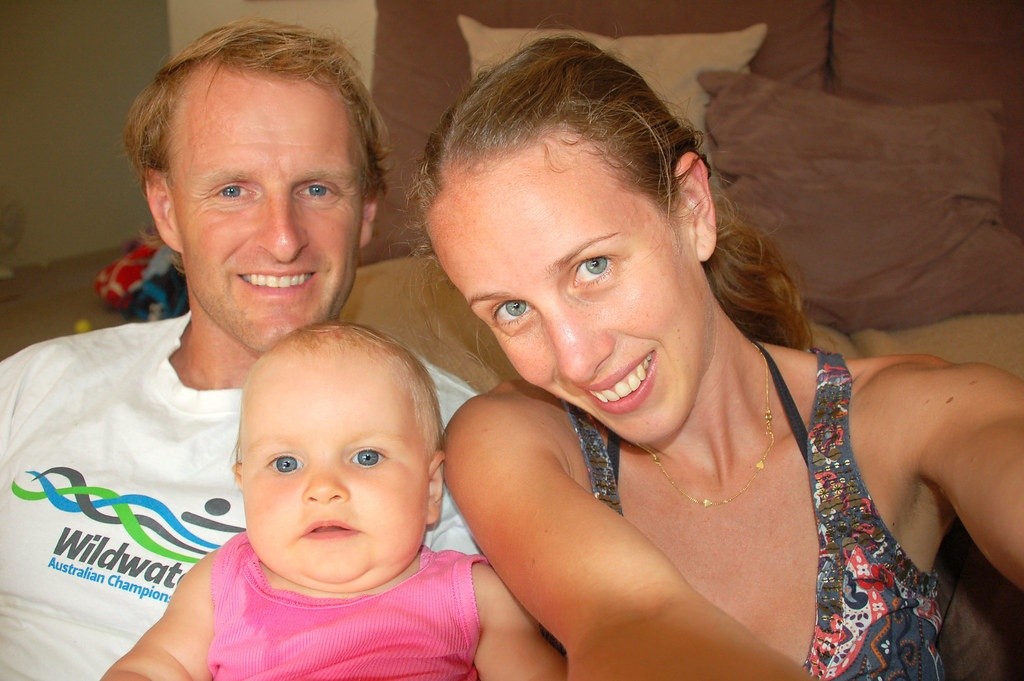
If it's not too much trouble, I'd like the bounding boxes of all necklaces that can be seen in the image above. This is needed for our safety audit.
[629,340,774,511]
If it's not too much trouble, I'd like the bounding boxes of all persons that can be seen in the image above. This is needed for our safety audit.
[0,19,488,681]
[99,321,568,681]
[418,37,1024,680]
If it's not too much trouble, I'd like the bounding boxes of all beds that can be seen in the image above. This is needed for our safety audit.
[339,1,1024,681]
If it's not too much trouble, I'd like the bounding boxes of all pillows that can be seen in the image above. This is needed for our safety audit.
[692,68,1024,329]
[353,0,832,267]
[456,15,771,197]
[830,1,1024,234]
